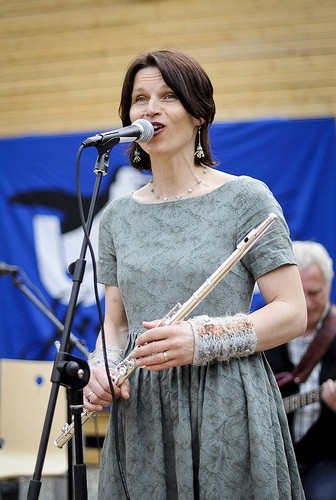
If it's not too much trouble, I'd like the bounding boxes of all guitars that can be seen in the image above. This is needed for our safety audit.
[276,371,335,413]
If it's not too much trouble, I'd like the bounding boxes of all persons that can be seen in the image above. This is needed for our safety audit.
[82,51,308,500]
[264,240,336,500]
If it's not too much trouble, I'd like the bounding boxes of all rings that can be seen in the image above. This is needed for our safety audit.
[163,351,169,362]
[86,390,95,400]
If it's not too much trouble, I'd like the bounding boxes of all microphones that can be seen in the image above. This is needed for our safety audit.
[81,118,154,148]
[0,261,19,277]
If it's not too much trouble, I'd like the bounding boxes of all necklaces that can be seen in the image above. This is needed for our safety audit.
[149,167,209,202]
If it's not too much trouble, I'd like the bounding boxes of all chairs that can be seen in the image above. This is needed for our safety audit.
[0,358,68,482]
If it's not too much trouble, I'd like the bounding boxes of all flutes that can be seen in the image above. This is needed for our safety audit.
[53,213,278,449]
